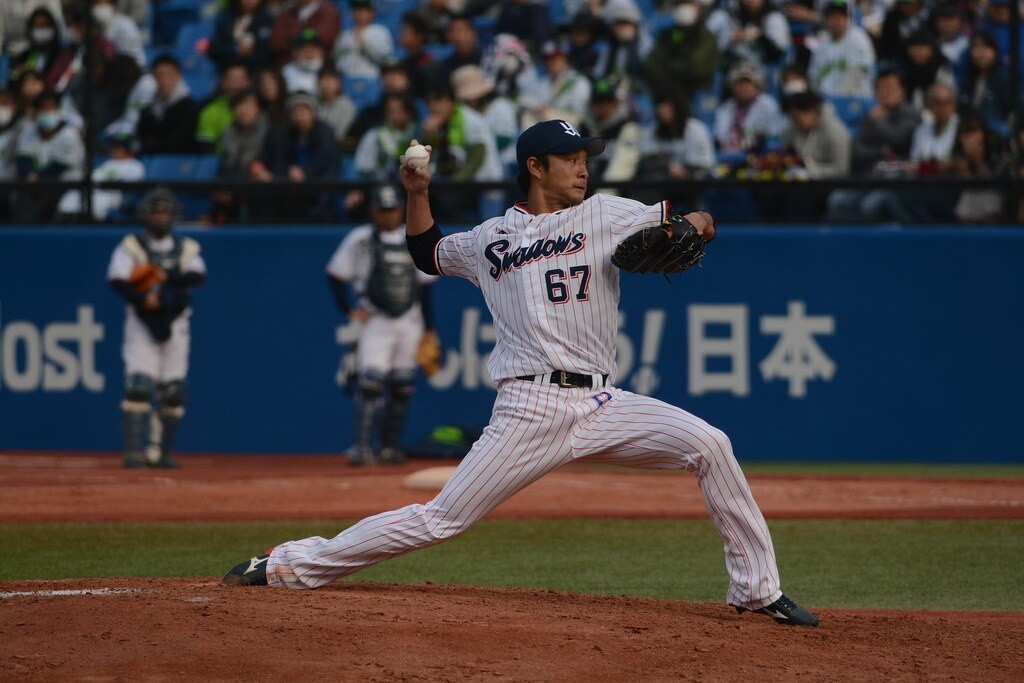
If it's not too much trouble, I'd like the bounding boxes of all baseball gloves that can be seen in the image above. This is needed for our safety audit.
[610,214,707,283]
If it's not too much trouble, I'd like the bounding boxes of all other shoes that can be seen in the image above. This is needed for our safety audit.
[122,449,143,468]
[347,443,374,467]
[146,450,179,468]
[378,446,406,465]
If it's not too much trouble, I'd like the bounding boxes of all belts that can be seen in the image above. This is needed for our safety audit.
[515,370,609,388]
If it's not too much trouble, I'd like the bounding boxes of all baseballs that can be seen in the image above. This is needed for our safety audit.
[405,145,430,169]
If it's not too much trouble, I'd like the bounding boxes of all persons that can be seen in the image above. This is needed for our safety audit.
[322,185,437,473]
[1,0,1024,227]
[220,119,820,630]
[105,189,207,470]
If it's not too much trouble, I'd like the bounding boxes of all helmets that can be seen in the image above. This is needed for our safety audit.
[138,187,177,239]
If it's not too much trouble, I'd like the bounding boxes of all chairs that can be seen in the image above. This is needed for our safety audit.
[1,1,878,223]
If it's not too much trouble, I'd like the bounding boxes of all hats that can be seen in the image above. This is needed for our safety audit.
[728,63,765,90]
[535,38,572,60]
[516,119,605,170]
[289,26,325,52]
[589,78,616,106]
[823,0,848,17]
[99,120,136,145]
[373,184,403,210]
[284,90,320,118]
[600,0,643,27]
[448,64,496,103]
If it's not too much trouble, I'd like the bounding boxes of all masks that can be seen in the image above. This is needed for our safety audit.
[670,2,699,27]
[614,27,636,42]
[0,3,115,130]
[781,78,808,96]
[500,54,522,76]
[298,57,325,72]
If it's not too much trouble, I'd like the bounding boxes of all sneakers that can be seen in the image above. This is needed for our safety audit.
[729,594,819,628]
[221,548,275,587]
